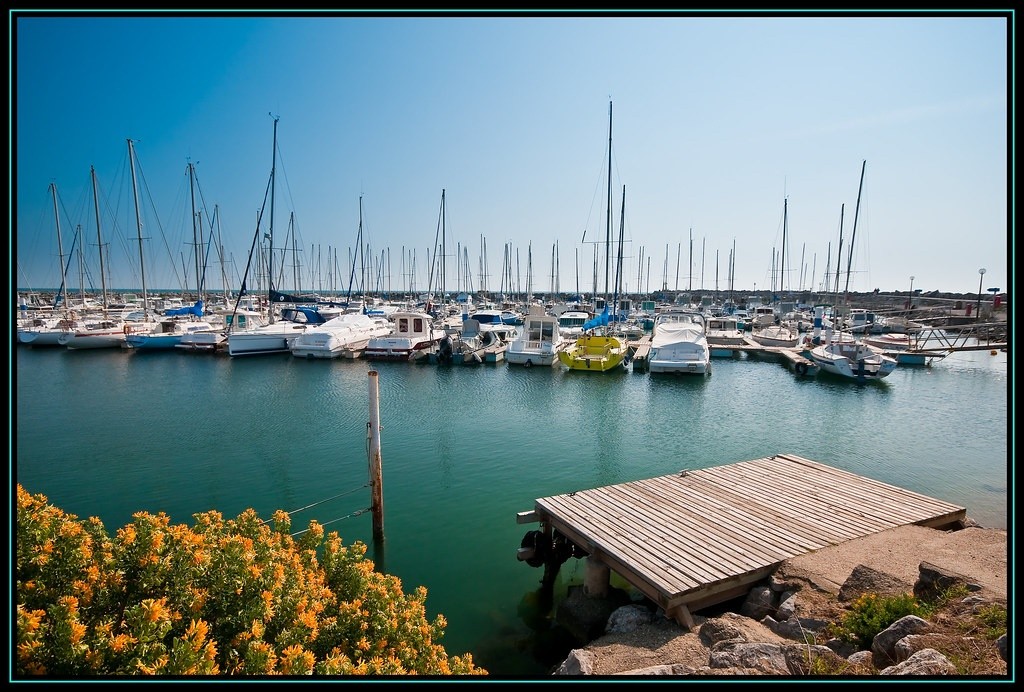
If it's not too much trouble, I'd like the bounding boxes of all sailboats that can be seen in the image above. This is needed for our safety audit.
[18,94,926,384]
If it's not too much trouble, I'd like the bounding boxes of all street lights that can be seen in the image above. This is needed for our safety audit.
[908,275,915,309]
[975,267,987,323]
